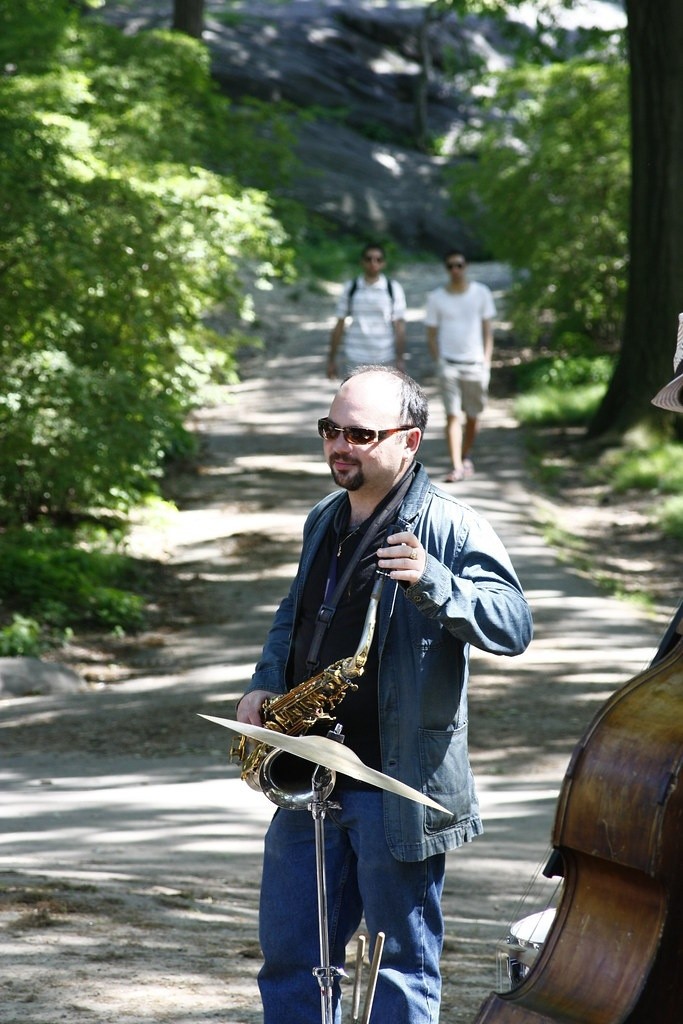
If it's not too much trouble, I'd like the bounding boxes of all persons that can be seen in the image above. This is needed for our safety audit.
[327,244,407,381]
[423,249,498,483]
[543,312,683,878]
[235,363,534,1023]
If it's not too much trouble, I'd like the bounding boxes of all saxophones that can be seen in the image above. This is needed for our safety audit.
[228,522,403,811]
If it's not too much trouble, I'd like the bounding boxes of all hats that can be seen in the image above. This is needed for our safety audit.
[651,312,683,412]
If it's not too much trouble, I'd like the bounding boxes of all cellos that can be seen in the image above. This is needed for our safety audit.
[469,592,683,1024]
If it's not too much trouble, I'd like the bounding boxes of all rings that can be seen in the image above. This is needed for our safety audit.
[410,548,418,560]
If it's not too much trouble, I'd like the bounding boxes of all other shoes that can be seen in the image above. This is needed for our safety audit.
[446,470,464,482]
[462,455,474,475]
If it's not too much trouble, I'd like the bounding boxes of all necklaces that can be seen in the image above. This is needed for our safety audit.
[337,526,360,557]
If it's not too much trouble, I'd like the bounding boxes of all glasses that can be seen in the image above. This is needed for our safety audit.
[364,255,384,264]
[447,262,467,269]
[318,417,415,445]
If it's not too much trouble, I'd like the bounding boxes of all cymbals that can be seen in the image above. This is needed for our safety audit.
[196,712,454,815]
